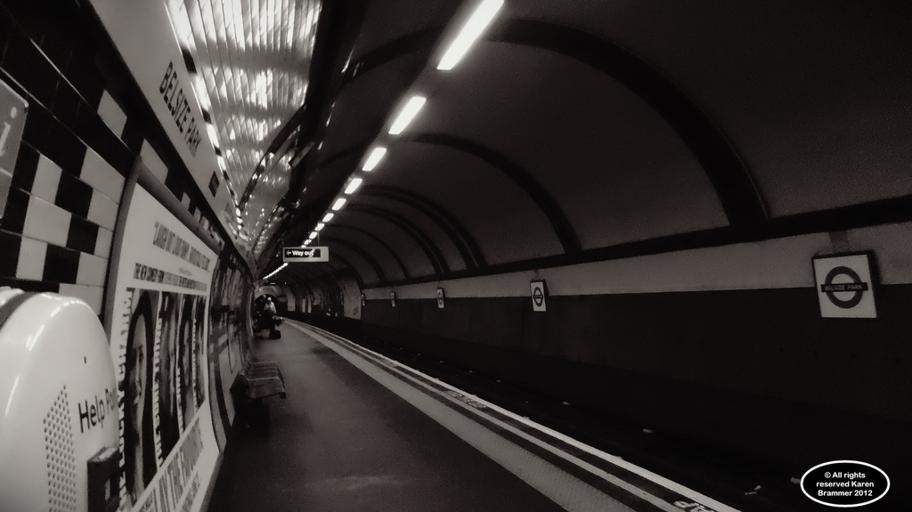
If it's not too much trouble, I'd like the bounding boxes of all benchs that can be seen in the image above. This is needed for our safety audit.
[240,364,286,400]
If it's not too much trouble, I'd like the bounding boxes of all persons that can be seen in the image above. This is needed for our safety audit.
[124,290,157,507]
[181,293,198,431]
[264,297,276,314]
[256,295,266,321]
